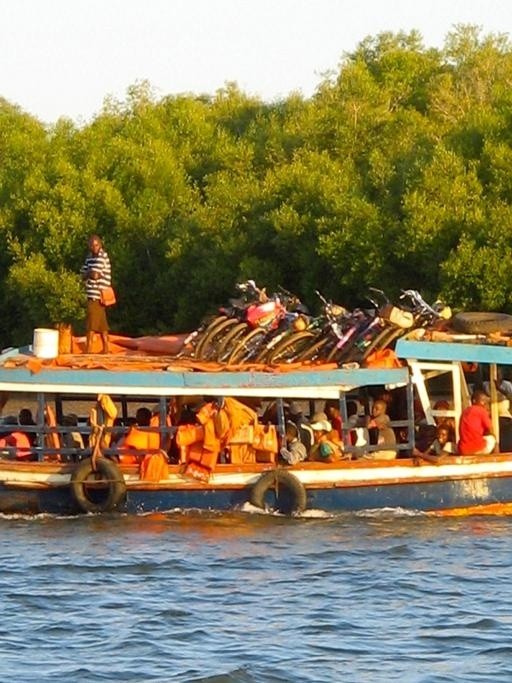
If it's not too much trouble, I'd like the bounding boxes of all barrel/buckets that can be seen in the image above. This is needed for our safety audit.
[33,329,59,358]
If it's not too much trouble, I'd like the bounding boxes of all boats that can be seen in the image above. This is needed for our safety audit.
[0,314,512,517]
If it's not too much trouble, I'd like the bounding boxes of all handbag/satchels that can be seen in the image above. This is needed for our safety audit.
[100,287,116,306]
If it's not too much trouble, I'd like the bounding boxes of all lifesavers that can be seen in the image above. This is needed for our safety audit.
[71,457,125,513]
[249,469,307,515]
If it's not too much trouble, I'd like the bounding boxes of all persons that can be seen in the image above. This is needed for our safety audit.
[0,362,511,476]
[78,233,118,355]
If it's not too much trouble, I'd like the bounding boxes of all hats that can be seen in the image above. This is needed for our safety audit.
[309,419,333,434]
[434,399,448,409]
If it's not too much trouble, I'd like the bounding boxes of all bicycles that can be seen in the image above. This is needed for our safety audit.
[179,280,453,368]
